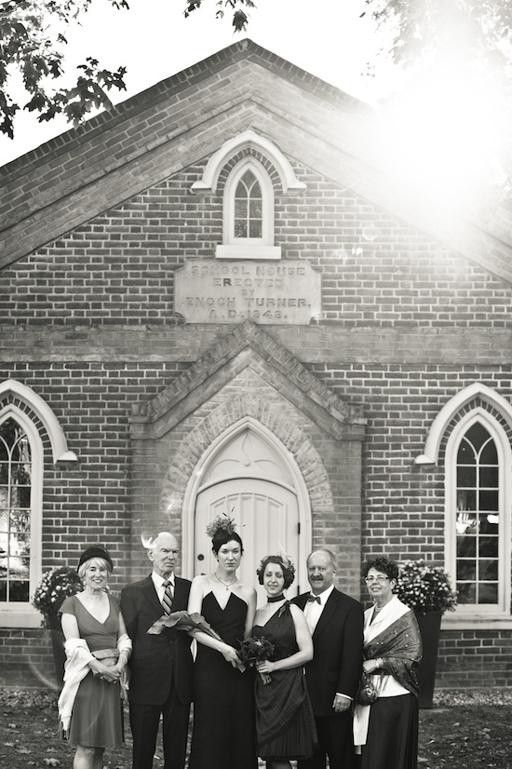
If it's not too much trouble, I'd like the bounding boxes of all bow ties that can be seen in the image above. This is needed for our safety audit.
[306,594,320,604]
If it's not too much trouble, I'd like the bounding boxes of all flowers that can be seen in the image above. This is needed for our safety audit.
[395,559,450,610]
[32,568,81,628]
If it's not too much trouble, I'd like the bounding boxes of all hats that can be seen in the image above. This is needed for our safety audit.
[76,547,113,574]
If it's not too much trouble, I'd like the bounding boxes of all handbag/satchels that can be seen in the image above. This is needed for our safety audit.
[356,672,378,705]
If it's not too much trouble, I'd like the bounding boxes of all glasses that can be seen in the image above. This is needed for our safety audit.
[364,576,394,586]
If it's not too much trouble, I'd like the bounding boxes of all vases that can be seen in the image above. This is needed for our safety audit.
[48,631,67,685]
[411,611,446,710]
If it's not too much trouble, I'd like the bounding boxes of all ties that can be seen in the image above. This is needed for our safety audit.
[162,582,173,616]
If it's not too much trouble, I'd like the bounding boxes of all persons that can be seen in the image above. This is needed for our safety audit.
[291,549,365,769]
[253,556,314,769]
[56,547,132,769]
[360,556,425,769]
[121,532,192,769]
[186,504,257,769]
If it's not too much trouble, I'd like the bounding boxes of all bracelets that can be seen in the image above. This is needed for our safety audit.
[376,657,383,668]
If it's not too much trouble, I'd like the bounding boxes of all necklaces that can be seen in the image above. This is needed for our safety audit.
[214,573,238,591]
[268,594,285,602]
[373,607,382,612]
